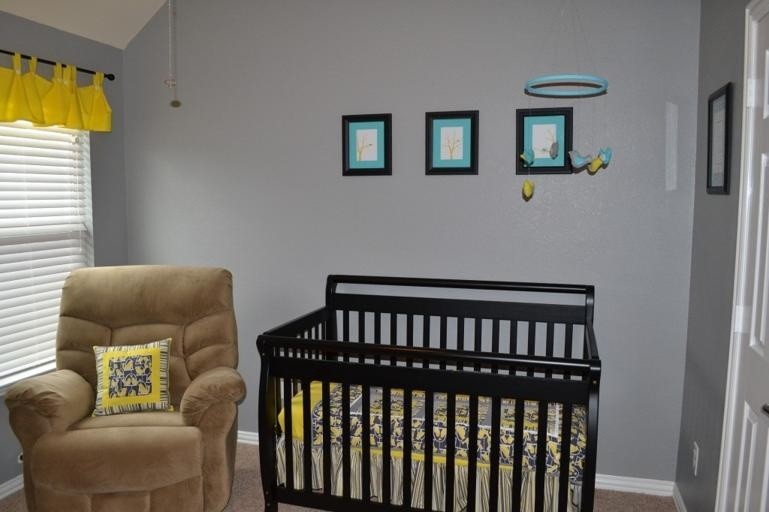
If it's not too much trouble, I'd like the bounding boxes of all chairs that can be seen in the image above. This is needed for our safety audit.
[1,265,247,512]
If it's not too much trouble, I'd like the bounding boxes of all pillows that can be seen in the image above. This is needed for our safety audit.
[89,336,174,419]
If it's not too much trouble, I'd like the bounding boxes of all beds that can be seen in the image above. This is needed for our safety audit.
[255,274,601,511]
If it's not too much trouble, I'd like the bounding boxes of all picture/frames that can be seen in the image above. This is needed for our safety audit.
[343,114,392,176]
[516,107,572,175]
[706,82,733,194]
[425,110,479,175]
